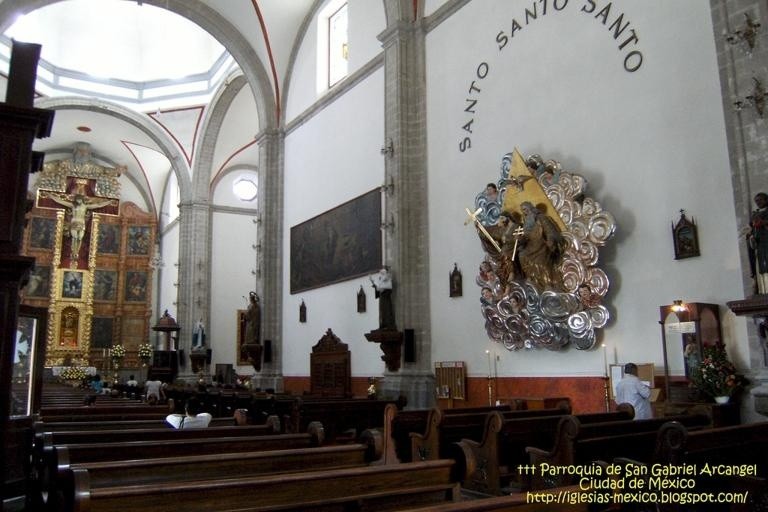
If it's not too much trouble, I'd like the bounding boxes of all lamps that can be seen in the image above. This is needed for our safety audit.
[671,300,682,312]
[233,174,258,202]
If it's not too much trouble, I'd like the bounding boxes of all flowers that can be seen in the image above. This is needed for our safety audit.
[137,343,154,359]
[60,366,89,380]
[688,340,749,396]
[111,344,126,358]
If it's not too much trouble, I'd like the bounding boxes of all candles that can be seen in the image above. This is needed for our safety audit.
[602,344,608,377]
[486,350,491,376]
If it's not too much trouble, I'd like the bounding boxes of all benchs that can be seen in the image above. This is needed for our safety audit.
[31,381,768,512]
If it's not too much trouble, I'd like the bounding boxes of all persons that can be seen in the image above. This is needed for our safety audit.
[89,373,103,393]
[746,192,767,296]
[45,193,118,264]
[244,294,260,344]
[614,362,654,420]
[196,371,275,399]
[110,389,119,399]
[81,393,96,408]
[143,374,167,405]
[94,320,112,346]
[683,335,699,388]
[25,268,44,297]
[66,272,80,292]
[80,373,93,390]
[102,223,118,251]
[127,285,145,301]
[476,160,603,320]
[146,393,158,405]
[165,396,213,429]
[131,232,146,254]
[35,220,49,248]
[128,271,145,287]
[97,270,113,300]
[126,374,137,387]
[192,316,206,348]
[158,379,169,388]
